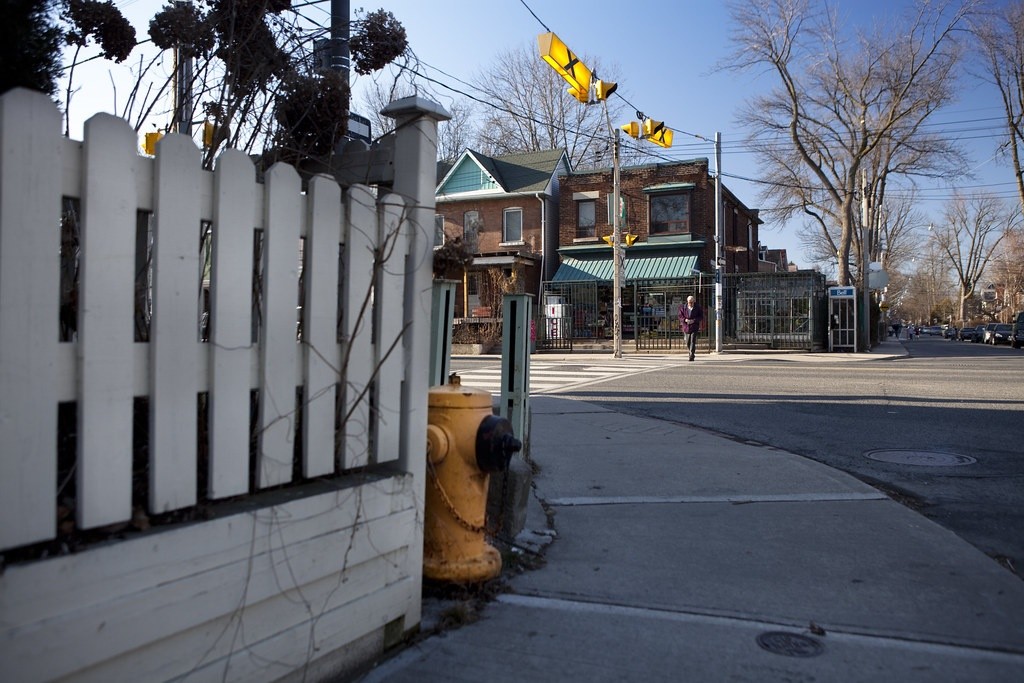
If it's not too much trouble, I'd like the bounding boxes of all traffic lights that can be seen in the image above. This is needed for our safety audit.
[602,234,614,248]
[648,119,664,136]
[597,79,618,100]
[203,118,230,150]
[625,234,639,246]
[621,121,640,138]
[567,86,588,103]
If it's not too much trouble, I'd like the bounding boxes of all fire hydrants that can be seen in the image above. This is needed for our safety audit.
[424,370,523,585]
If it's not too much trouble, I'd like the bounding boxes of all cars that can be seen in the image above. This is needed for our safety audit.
[901,309,1024,349]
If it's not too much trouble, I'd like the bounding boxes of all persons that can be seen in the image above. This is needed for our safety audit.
[894,321,903,339]
[593,311,610,337]
[909,327,913,340]
[678,296,703,361]
[913,326,920,341]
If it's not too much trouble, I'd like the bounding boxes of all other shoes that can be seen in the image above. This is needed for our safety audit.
[689,356,694,361]
